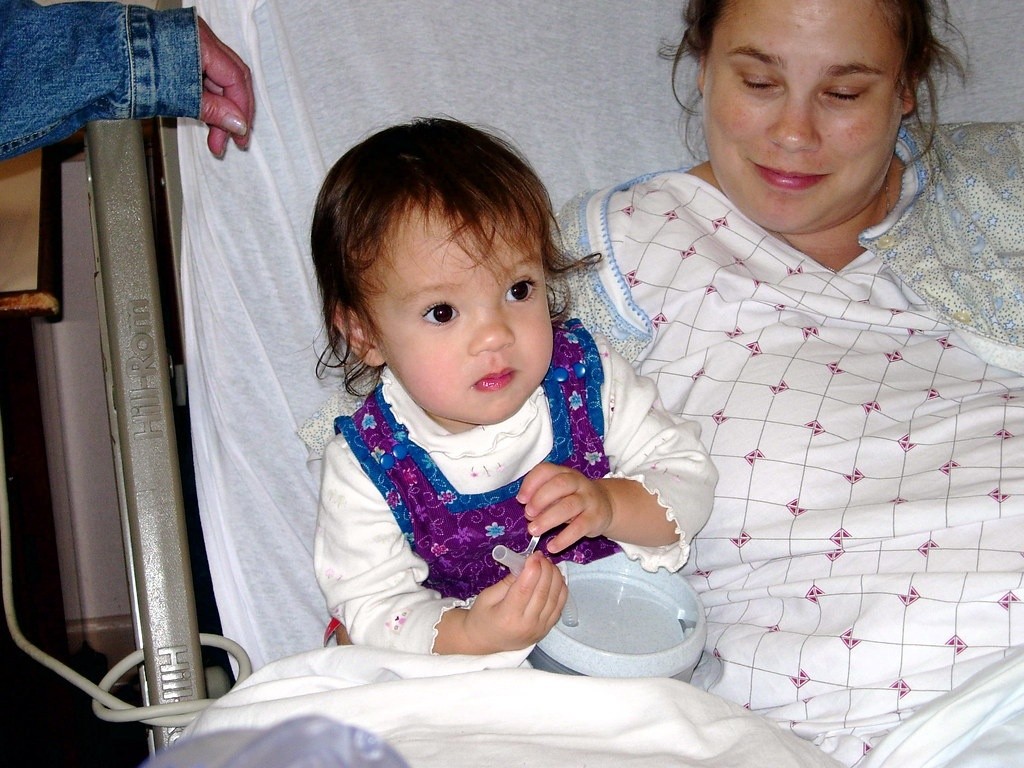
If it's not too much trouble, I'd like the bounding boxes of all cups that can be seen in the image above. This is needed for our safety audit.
[524,550,707,684]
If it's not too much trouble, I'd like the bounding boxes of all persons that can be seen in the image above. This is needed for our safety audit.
[323,0,1024,768]
[1,0,253,162]
[308,114,718,654]
